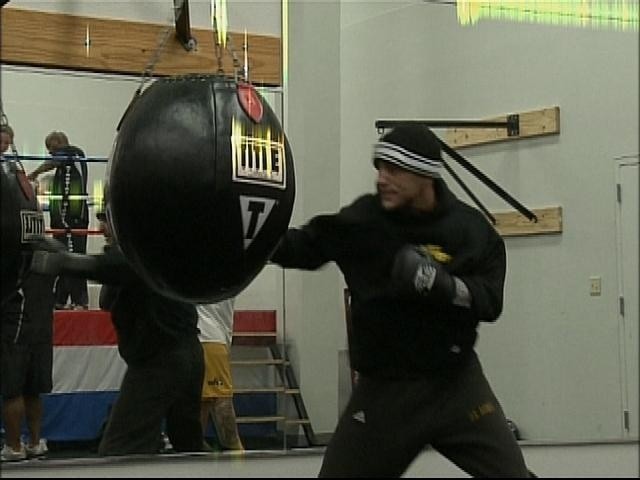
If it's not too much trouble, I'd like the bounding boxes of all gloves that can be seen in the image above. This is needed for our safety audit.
[29,234,69,277]
[392,243,456,304]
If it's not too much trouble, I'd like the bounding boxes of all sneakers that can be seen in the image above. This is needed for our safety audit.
[0,441,27,462]
[25,438,48,460]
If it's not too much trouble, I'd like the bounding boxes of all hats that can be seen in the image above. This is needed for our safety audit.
[373,122,445,181]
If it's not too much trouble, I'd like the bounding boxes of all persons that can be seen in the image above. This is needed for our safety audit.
[266,120,539,478]
[30,205,206,455]
[196,296,246,451]
[26,130,90,311]
[1,153,45,463]
[0,123,15,178]
